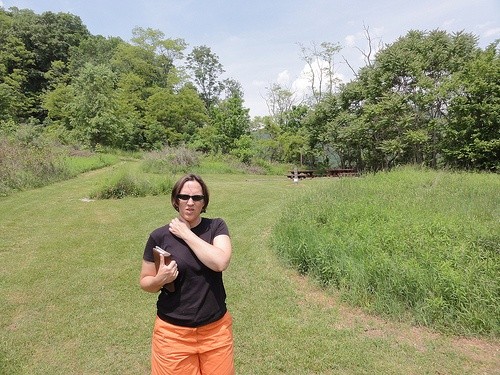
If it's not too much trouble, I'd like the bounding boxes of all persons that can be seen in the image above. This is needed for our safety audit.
[140,174,236,375]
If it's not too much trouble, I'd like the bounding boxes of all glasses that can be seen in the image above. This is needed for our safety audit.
[176,192,206,201]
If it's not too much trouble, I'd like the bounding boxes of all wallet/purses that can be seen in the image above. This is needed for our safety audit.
[152,245,175,293]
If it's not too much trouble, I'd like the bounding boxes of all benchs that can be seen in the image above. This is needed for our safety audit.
[287,169,316,179]
[323,168,360,177]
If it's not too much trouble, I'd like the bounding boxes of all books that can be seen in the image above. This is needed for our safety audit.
[153,246,175,292]
